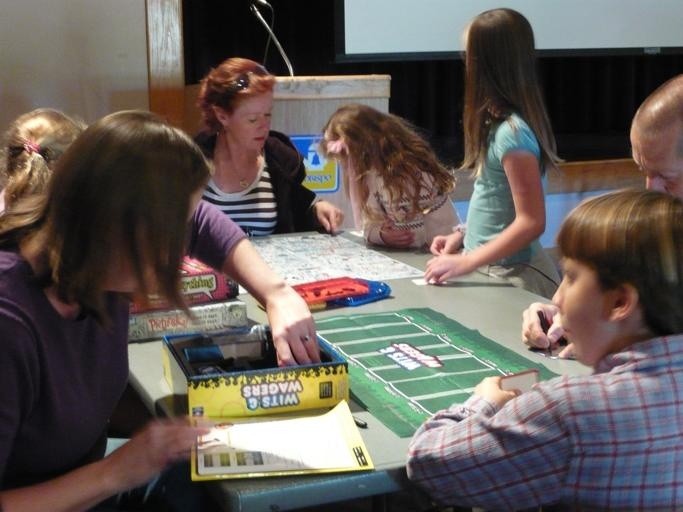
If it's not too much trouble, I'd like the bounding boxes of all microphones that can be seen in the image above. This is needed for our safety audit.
[251,0,272,11]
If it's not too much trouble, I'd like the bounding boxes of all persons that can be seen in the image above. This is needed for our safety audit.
[319,104,463,250]
[425,8,562,301]
[186,57,345,238]
[1,108,322,512]
[521,74,683,358]
[406,188,682,511]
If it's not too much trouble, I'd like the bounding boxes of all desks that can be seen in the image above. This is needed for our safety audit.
[125,222,599,511]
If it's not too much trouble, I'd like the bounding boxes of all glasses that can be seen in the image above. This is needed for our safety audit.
[221,65,273,108]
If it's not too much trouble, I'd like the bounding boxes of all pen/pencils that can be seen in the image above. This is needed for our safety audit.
[353,416,366,428]
[539,310,553,357]
